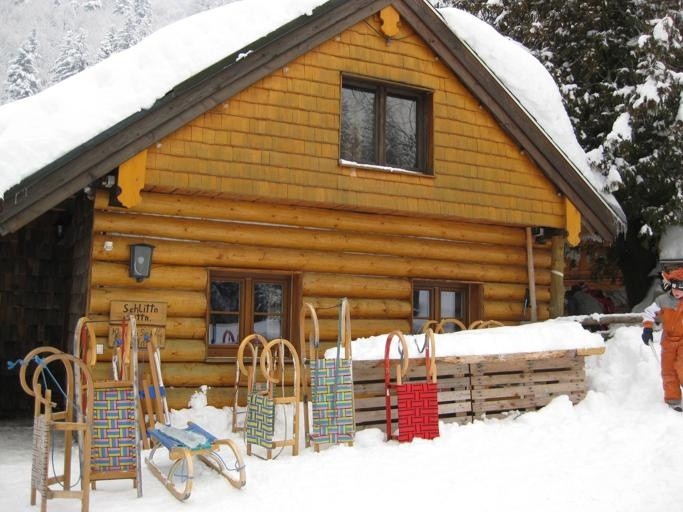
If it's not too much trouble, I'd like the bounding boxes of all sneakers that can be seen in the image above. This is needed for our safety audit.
[670,404,682,411]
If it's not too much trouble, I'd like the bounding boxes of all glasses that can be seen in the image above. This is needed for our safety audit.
[671,282,683,290]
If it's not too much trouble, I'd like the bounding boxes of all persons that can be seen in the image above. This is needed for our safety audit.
[640,268,683,411]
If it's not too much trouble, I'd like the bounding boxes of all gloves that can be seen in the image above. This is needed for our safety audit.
[642,328,653,345]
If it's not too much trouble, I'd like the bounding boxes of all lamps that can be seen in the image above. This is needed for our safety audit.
[128,243,156,283]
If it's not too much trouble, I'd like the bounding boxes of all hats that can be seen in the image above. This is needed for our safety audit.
[669,268,683,280]
